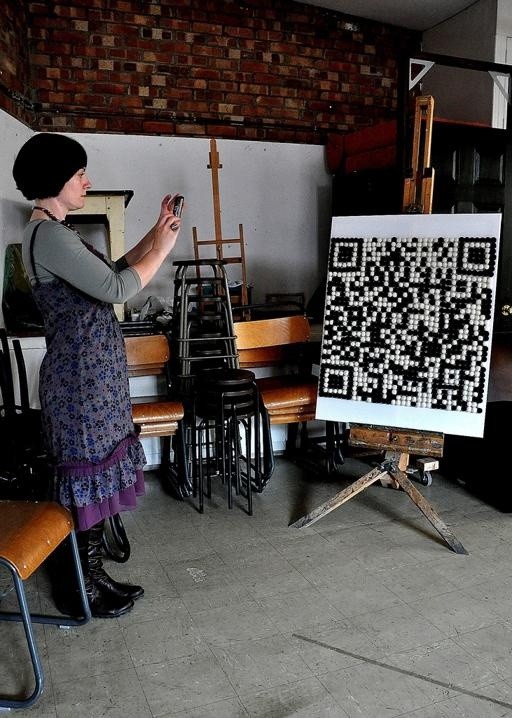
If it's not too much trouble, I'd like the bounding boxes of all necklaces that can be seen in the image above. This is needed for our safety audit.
[33,206,81,235]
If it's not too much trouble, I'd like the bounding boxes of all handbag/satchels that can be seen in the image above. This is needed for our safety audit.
[1,404,58,504]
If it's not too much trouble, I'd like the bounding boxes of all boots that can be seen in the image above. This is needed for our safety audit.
[53,530,134,618]
[89,526,145,600]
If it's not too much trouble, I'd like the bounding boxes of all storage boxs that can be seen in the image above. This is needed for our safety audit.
[325,115,492,175]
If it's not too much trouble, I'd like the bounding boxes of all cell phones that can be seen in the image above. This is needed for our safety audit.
[171,196,184,218]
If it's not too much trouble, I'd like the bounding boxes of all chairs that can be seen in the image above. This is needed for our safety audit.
[0,499,92,707]
[119,322,185,499]
[231,301,341,482]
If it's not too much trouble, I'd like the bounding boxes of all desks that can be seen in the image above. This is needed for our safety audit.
[64,190,134,322]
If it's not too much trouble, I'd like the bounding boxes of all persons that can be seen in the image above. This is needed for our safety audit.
[12,133,188,618]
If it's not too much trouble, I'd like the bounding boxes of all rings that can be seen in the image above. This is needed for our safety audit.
[169,222,179,231]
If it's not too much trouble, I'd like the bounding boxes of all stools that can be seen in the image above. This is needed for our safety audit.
[174,259,263,516]
